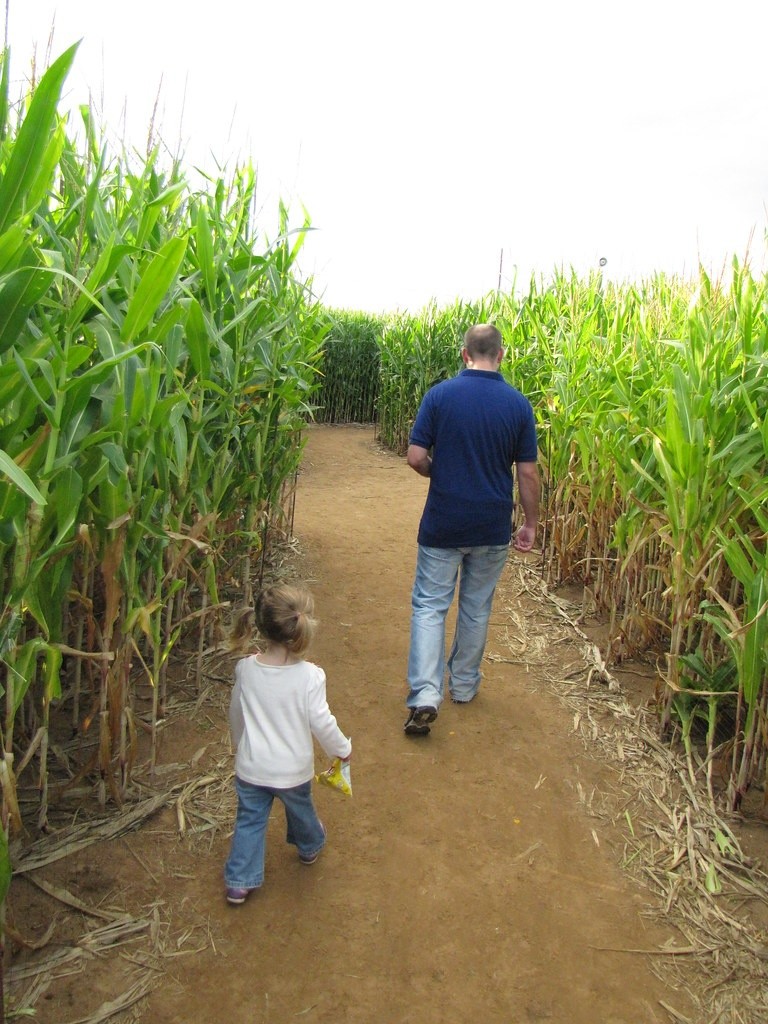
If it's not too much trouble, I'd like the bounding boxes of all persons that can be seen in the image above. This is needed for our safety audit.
[226,584,353,907]
[404,325,544,735]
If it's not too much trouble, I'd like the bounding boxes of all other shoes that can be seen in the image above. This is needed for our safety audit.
[226,887,253,903]
[403,706,437,736]
[299,818,325,864]
